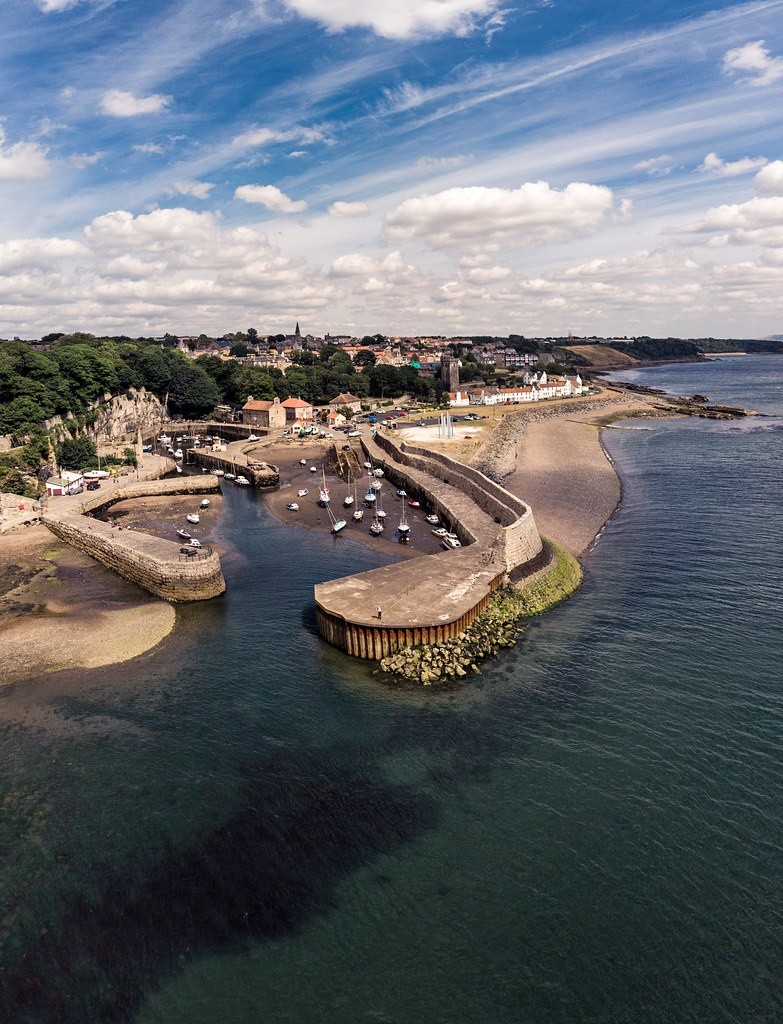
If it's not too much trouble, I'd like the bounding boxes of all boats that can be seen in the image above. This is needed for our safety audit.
[372,469,384,477]
[333,520,346,532]
[224,474,236,480]
[185,538,202,548]
[397,490,407,496]
[202,499,209,507]
[371,522,383,534]
[298,489,309,498]
[287,503,299,512]
[235,478,249,484]
[176,530,191,539]
[310,467,316,471]
[377,510,387,517]
[364,462,372,469]
[425,515,439,525]
[300,460,307,464]
[187,515,200,524]
[442,533,461,550]
[320,494,330,503]
[365,493,377,503]
[354,510,364,520]
[398,524,410,532]
[345,495,354,504]
[432,527,448,537]
[409,500,420,509]
[371,480,382,492]
[210,468,225,476]
[143,433,222,459]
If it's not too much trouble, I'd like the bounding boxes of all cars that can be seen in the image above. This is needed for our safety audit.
[563,390,599,400]
[465,413,480,421]
[248,405,411,442]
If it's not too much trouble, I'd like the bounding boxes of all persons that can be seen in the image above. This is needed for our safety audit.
[376,606,382,619]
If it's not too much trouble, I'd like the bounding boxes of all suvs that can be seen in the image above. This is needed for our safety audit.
[87,481,100,491]
